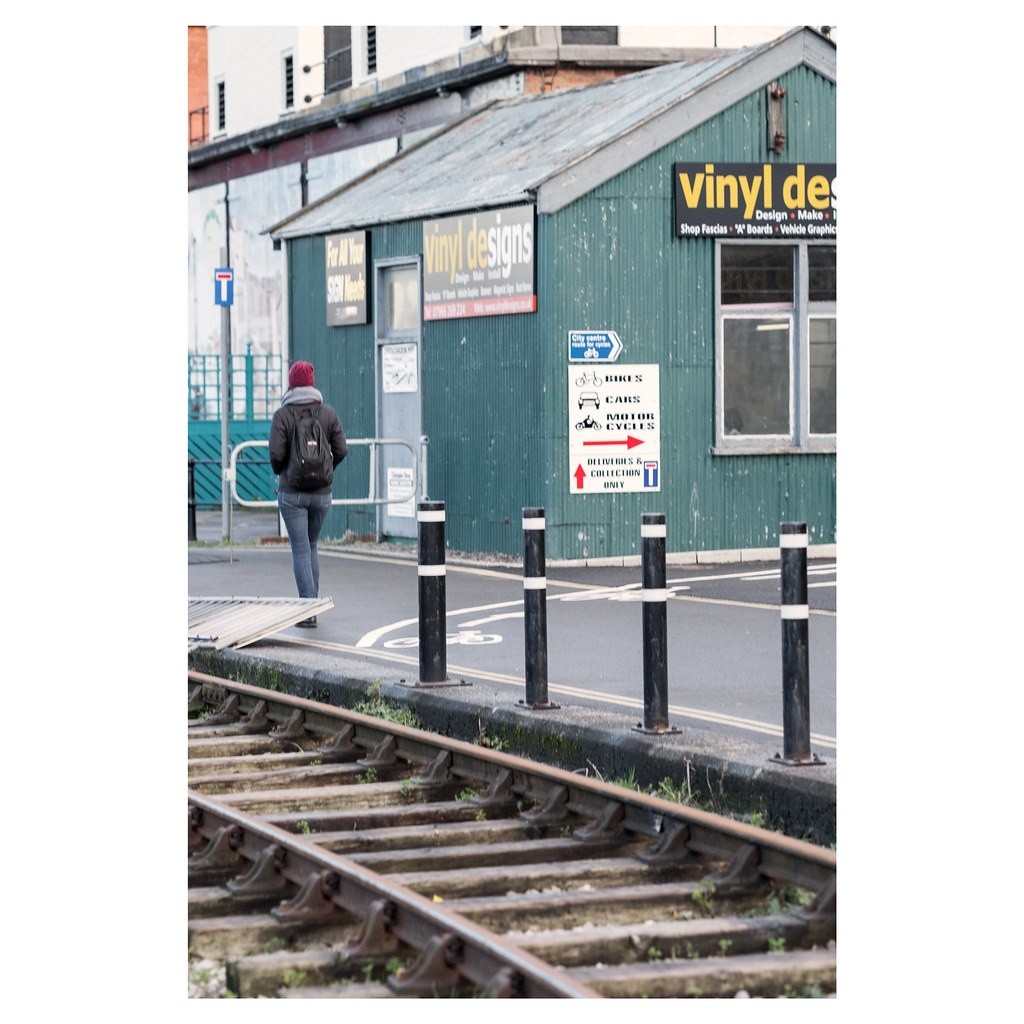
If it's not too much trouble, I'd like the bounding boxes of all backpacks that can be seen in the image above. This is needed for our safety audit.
[287,404,333,491]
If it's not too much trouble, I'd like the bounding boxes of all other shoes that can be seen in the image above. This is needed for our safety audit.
[295,616,317,627]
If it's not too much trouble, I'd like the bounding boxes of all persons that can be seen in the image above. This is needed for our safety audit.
[269,360,348,627]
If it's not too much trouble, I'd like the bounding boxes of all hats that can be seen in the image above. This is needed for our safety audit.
[288,360,315,389]
